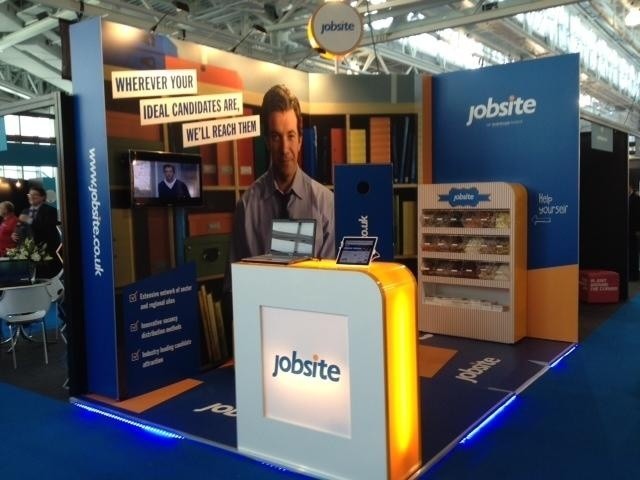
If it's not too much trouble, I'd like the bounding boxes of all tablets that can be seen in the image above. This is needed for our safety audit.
[336,236,378,265]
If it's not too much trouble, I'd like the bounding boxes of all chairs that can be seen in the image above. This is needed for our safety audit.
[0,277,58,371]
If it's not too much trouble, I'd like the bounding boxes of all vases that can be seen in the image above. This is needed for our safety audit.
[28,262,41,284]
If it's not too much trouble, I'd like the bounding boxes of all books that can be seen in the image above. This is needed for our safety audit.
[199,284,231,363]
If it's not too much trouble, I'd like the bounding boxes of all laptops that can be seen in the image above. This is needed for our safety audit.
[240,219,317,265]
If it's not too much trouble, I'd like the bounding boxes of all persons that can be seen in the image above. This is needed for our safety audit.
[222,82,335,354]
[157,164,191,199]
[0,186,57,259]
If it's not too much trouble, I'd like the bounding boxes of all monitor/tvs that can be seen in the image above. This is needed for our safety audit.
[128,148,204,208]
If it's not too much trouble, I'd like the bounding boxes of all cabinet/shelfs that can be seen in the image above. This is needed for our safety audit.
[98,17,431,401]
[417,181,529,343]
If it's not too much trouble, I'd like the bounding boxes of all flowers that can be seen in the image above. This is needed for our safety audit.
[6,235,55,262]
